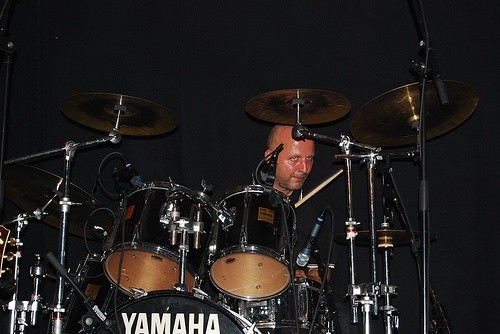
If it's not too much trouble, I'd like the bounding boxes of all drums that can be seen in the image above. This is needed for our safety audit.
[208,183,298,302]
[79,285,264,334]
[102,179,215,296]
[239,277,325,332]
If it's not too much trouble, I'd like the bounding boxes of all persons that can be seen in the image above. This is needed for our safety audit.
[211,124,338,289]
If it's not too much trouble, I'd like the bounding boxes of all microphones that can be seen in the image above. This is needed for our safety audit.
[296,208,328,267]
[122,155,141,187]
[264,148,279,188]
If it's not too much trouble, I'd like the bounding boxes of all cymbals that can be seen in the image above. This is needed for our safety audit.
[1,160,117,244]
[332,226,440,248]
[245,87,352,125]
[62,91,187,137]
[350,79,479,150]
